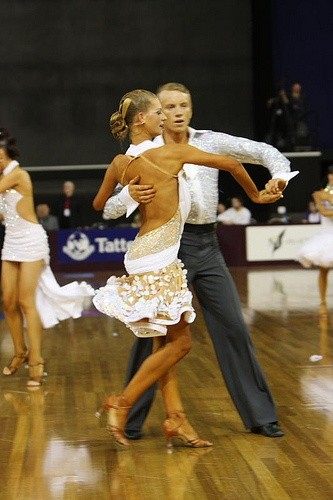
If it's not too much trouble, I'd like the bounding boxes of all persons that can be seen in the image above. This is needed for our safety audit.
[302,201,321,224]
[217,201,227,215]
[290,167,333,320]
[102,83,300,438]
[92,88,282,449]
[0,130,95,388]
[280,83,304,151]
[57,180,82,228]
[216,197,251,226]
[35,205,57,230]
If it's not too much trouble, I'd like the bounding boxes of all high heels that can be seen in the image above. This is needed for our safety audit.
[24,359,48,387]
[96,394,131,449]
[3,347,29,376]
[162,410,214,448]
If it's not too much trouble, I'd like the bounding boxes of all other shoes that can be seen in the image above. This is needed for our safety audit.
[251,421,285,437]
[123,429,144,440]
